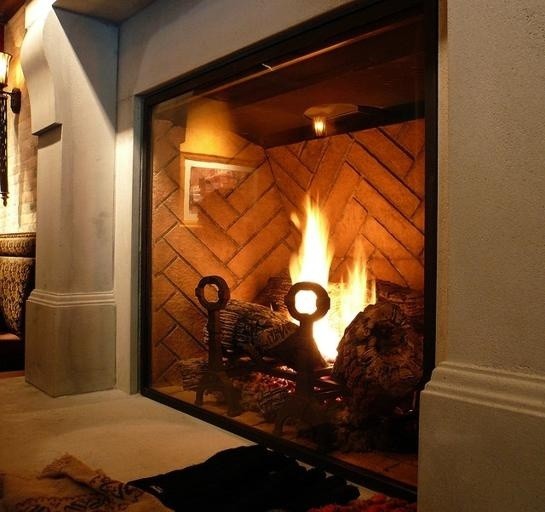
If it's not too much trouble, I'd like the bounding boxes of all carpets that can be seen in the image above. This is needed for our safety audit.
[2,450,172,512]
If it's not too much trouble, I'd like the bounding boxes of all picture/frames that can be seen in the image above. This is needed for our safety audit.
[178,151,260,228]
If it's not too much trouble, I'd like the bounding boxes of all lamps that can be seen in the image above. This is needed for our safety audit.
[314,116,327,137]
[0,48,22,111]
